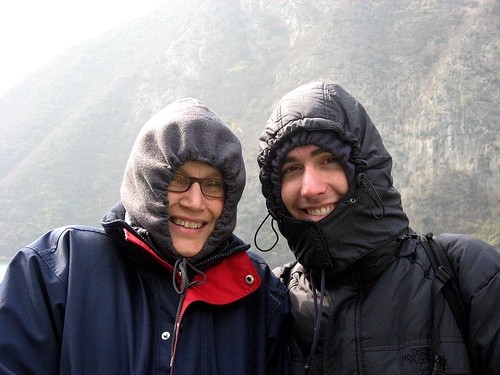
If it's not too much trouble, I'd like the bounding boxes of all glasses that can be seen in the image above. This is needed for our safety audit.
[166,171,228,200]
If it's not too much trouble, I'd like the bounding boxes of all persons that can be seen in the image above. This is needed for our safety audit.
[253,83,500,375]
[0,96,292,375]
